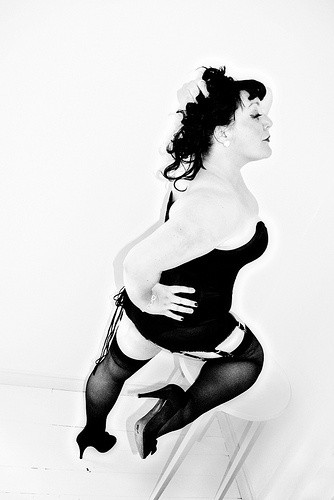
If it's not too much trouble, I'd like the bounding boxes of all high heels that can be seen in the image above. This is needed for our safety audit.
[75,429,117,459]
[135,385,184,457]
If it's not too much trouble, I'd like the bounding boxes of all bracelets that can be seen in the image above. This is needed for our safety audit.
[141,287,158,313]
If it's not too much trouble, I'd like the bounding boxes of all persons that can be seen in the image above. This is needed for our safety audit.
[74,63,276,466]
